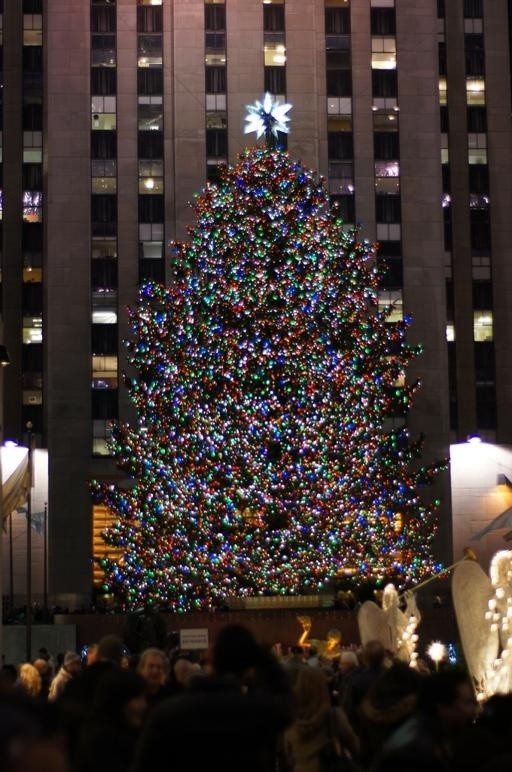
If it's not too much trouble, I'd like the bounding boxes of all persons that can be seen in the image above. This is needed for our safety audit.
[1,615,511,772]
